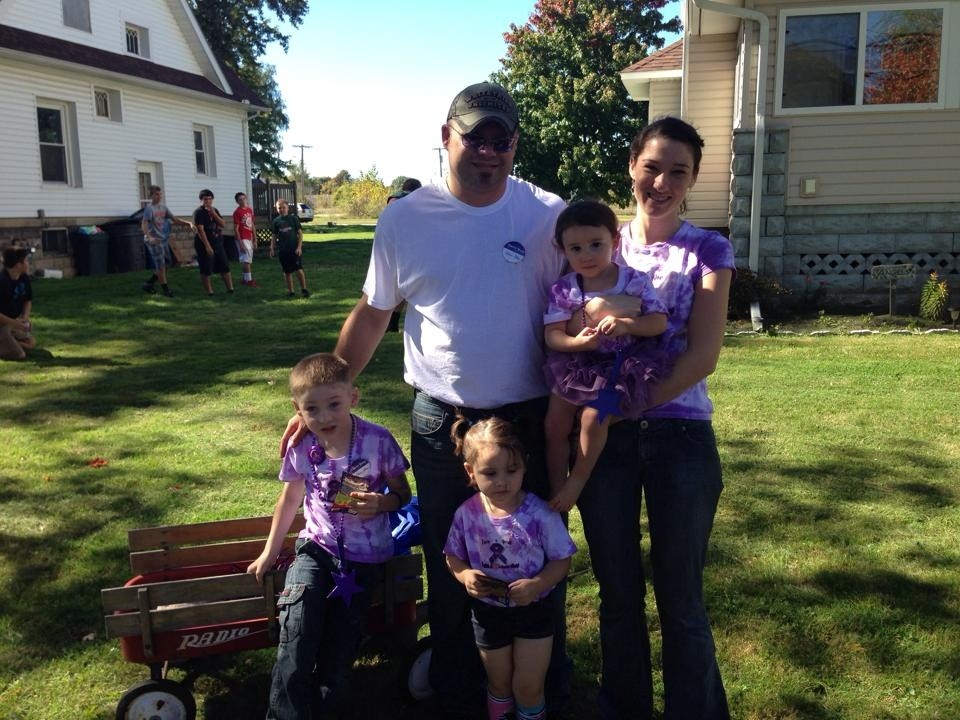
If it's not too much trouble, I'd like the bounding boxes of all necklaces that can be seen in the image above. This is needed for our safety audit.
[309,412,356,571]
[579,274,586,329]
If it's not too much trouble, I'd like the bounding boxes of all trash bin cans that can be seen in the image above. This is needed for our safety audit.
[102,219,148,273]
[71,224,108,275]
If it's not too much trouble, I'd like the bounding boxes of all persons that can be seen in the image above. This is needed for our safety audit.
[193,189,234,296]
[248,83,737,720]
[271,199,311,298]
[0,247,36,361]
[385,179,422,333]
[143,186,197,297]
[233,192,258,288]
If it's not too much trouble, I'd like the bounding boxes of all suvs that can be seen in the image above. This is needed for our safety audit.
[289,203,314,222]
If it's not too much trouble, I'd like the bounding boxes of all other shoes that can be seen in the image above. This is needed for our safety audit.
[302,288,308,297]
[142,284,155,294]
[162,291,173,297]
[240,279,256,287]
[285,293,296,299]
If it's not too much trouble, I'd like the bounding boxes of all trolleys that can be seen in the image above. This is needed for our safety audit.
[99,510,435,720]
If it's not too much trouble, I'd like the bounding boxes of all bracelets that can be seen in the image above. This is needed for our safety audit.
[386,491,402,512]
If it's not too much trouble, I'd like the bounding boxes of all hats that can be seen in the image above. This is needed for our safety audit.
[448,81,519,136]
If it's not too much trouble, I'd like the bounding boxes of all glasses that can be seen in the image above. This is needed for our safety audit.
[447,125,517,153]
